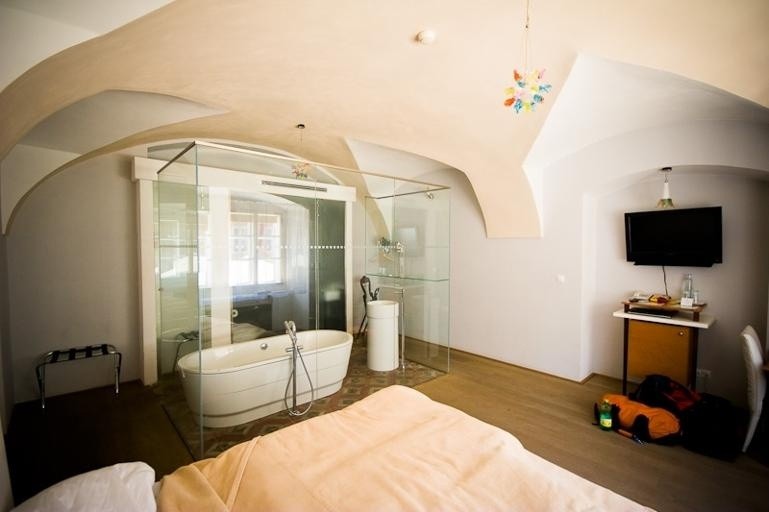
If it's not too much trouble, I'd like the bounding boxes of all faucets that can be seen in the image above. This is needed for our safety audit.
[374,288,379,299]
[286,321,296,346]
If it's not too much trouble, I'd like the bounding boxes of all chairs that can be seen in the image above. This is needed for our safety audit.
[356,277,379,337]
[739,325,769,452]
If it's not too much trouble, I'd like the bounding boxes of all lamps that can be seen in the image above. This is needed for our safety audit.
[655,167,674,210]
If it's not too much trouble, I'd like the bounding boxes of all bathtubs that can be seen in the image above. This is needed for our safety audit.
[177,329,353,429]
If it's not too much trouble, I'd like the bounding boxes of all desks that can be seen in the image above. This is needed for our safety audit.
[613,307,717,397]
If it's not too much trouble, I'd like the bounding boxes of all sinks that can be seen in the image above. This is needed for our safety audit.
[366,300,399,320]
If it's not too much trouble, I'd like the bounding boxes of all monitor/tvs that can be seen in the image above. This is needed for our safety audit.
[625,206,722,267]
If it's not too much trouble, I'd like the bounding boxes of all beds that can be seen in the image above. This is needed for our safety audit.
[10,384,658,512]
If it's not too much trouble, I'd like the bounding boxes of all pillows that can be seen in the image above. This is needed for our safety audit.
[12,462,157,512]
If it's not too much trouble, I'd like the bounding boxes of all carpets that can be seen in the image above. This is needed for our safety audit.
[159,346,447,462]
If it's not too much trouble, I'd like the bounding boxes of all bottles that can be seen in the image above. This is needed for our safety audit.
[599,398,613,431]
[682,274,693,298]
[691,289,699,304]
